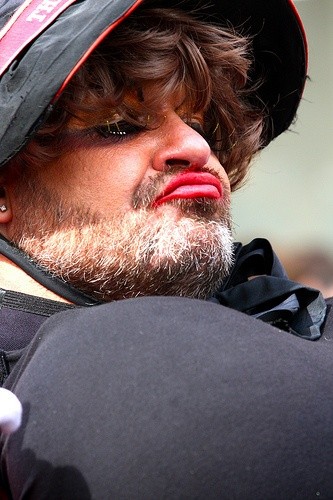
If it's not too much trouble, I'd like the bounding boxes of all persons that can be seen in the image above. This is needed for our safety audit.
[0,0,333,500]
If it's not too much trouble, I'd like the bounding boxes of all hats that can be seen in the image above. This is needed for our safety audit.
[0,0,309,169]
